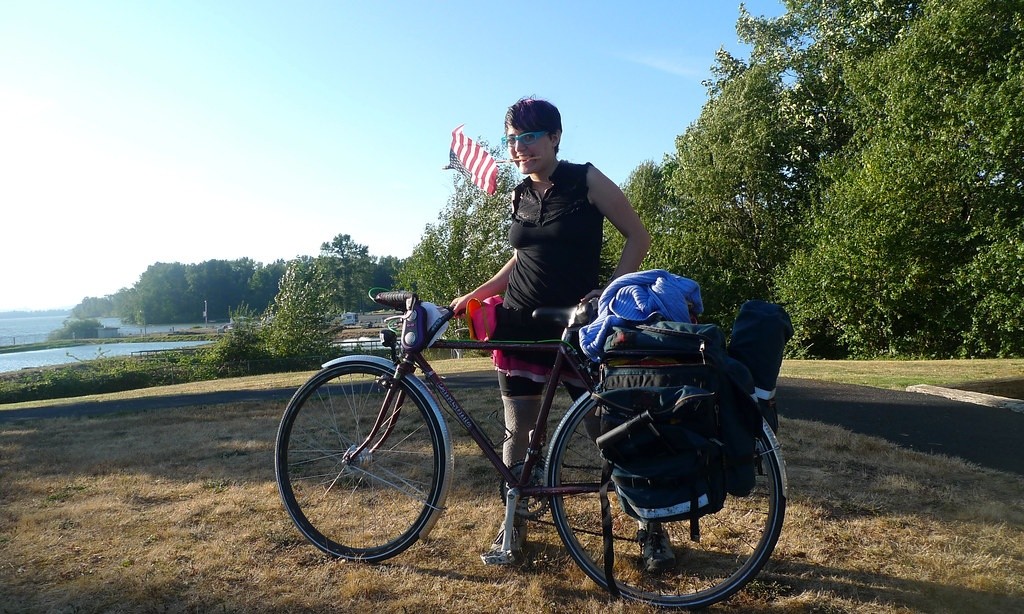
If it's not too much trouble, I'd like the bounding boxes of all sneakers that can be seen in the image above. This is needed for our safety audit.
[489,520,528,557]
[638,529,676,573]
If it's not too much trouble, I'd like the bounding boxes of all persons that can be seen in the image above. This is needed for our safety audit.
[450,101,677,572]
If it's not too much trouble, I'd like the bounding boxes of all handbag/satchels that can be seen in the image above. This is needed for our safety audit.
[591,320,759,522]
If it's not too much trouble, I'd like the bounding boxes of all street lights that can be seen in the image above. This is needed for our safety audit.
[204,300,207,328]
[140,310,146,335]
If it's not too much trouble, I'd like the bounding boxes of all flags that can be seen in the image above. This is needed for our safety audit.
[449,125,498,195]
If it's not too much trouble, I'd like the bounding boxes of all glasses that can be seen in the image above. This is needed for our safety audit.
[502,130,550,147]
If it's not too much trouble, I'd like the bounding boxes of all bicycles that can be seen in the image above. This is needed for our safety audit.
[274,290,790,609]
